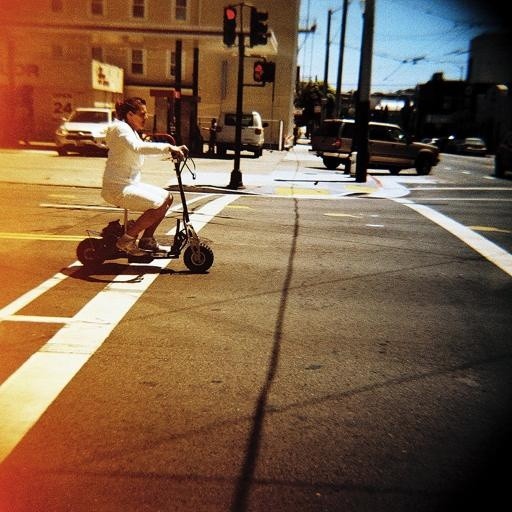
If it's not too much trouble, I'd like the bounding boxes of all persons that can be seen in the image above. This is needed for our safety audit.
[101,97,189,257]
[201,118,218,154]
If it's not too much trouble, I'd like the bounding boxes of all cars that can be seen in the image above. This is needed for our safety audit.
[493,127,512,179]
[389,128,490,156]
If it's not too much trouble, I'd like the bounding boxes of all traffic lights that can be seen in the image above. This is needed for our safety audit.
[252,60,276,83]
[220,4,237,47]
[248,2,270,49]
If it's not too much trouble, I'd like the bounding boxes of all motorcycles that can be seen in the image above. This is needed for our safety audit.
[72,143,216,274]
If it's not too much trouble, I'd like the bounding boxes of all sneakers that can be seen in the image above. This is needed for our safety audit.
[116,235,146,258]
[137,237,163,250]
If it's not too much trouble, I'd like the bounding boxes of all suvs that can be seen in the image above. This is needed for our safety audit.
[214,108,270,157]
[52,106,117,159]
[314,113,443,177]
